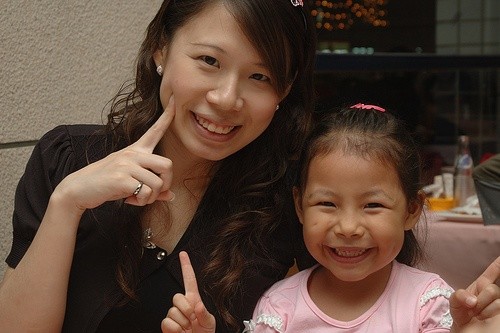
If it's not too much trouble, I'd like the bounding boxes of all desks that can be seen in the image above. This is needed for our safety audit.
[411,206,500,290]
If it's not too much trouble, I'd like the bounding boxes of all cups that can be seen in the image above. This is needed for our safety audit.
[433,168,455,199]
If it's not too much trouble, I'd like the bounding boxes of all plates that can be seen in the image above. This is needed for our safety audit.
[437,210,483,222]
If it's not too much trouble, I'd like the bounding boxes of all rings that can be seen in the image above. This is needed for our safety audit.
[132,179,144,197]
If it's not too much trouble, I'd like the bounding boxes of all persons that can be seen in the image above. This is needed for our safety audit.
[160,101,500,333]
[0,0,319,333]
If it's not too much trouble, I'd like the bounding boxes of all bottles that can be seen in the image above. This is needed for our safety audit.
[454,135,473,201]
